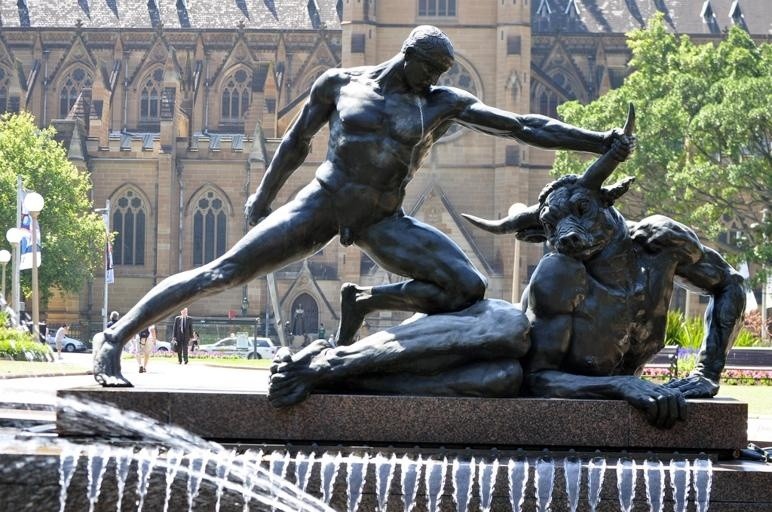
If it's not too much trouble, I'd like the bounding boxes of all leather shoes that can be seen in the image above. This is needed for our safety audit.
[185,359,188,364]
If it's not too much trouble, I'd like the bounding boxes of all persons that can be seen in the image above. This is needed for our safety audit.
[286,302,310,348]
[329,334,336,347]
[317,322,325,339]
[92,25,635,389]
[55,324,68,359]
[173,308,192,364]
[191,332,199,352]
[106,310,119,329]
[241,297,248,316]
[133,323,157,373]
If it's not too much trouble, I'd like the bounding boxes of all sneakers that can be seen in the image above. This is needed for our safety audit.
[139,365,143,373]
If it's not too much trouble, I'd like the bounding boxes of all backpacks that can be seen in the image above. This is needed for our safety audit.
[139,328,150,345]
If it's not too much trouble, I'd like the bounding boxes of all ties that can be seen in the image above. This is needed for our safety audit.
[183,317,185,333]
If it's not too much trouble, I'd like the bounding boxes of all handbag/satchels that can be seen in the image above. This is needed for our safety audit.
[171,341,178,352]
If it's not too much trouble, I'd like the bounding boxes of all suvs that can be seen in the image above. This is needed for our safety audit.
[190,334,277,360]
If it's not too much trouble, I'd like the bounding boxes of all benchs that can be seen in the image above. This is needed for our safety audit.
[640,345,679,380]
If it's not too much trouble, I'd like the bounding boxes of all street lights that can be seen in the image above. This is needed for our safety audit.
[0,251,11,312]
[7,228,21,324]
[24,193,44,342]
[508,203,526,304]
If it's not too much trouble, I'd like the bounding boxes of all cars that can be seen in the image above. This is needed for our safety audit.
[47,333,87,352]
[93,329,171,351]
